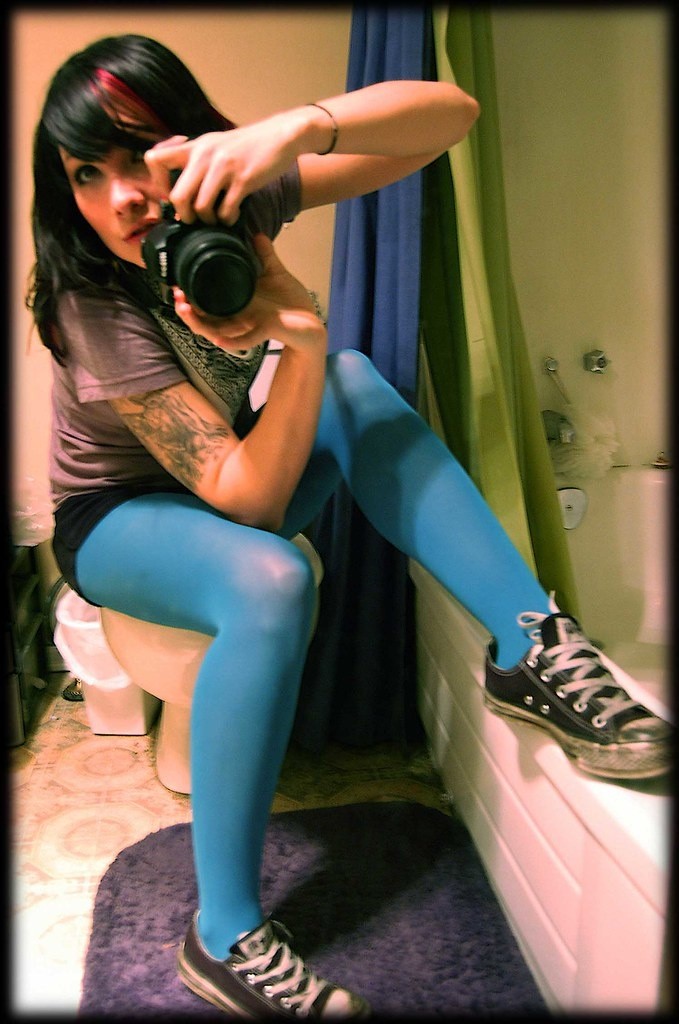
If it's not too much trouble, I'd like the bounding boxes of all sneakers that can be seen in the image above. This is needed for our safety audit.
[175,909,354,1020]
[481,590,671,780]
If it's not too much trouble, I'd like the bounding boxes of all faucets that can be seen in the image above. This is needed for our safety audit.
[539,408,574,445]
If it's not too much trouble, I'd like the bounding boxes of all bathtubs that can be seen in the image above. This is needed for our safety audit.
[405,451,678,1020]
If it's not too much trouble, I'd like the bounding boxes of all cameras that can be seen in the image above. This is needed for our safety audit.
[139,194,259,319]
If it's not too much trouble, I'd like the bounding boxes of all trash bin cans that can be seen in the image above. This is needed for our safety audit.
[51,587,162,737]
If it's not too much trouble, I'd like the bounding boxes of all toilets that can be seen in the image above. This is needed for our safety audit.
[104,293,329,795]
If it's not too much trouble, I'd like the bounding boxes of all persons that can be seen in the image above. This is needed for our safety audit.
[26,33,672,1016]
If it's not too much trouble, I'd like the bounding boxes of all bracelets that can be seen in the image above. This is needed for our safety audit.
[309,100,341,160]
